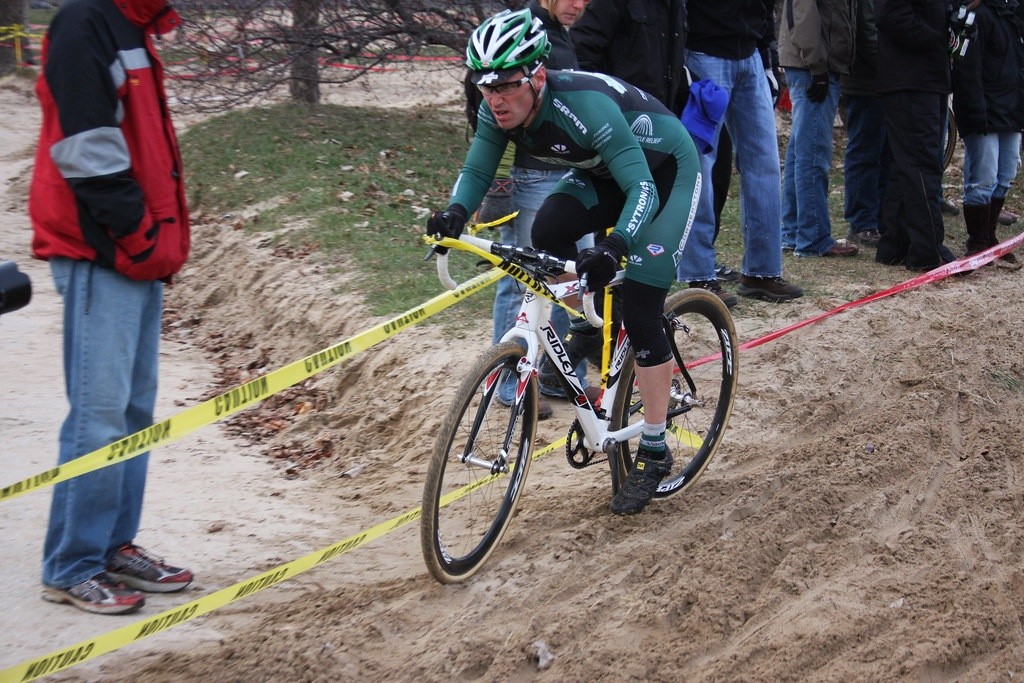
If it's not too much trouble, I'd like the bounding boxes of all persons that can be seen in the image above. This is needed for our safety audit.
[428,8,703,516]
[465,0,602,419]
[569,0,1024,370]
[29,0,193,613]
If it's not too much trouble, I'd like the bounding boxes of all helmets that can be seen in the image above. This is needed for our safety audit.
[464,8,551,84]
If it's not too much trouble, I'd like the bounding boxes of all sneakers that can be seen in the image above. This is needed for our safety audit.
[42,570,147,614]
[689,280,735,306]
[610,442,674,516]
[736,275,803,303]
[104,540,194,592]
[539,326,602,387]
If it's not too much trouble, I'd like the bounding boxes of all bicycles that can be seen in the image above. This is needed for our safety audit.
[421,224,744,585]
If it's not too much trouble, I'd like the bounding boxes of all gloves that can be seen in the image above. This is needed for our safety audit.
[575,233,628,292]
[427,203,468,256]
[807,73,829,104]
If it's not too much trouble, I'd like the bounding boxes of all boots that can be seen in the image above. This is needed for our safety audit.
[962,197,1015,276]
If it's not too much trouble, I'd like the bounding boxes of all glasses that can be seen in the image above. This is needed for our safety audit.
[475,58,545,96]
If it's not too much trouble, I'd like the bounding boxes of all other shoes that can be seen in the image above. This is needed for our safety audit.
[494,391,555,420]
[827,243,860,256]
[542,387,600,403]
[714,262,736,279]
[848,230,881,245]
[998,213,1016,225]
[941,200,960,216]
